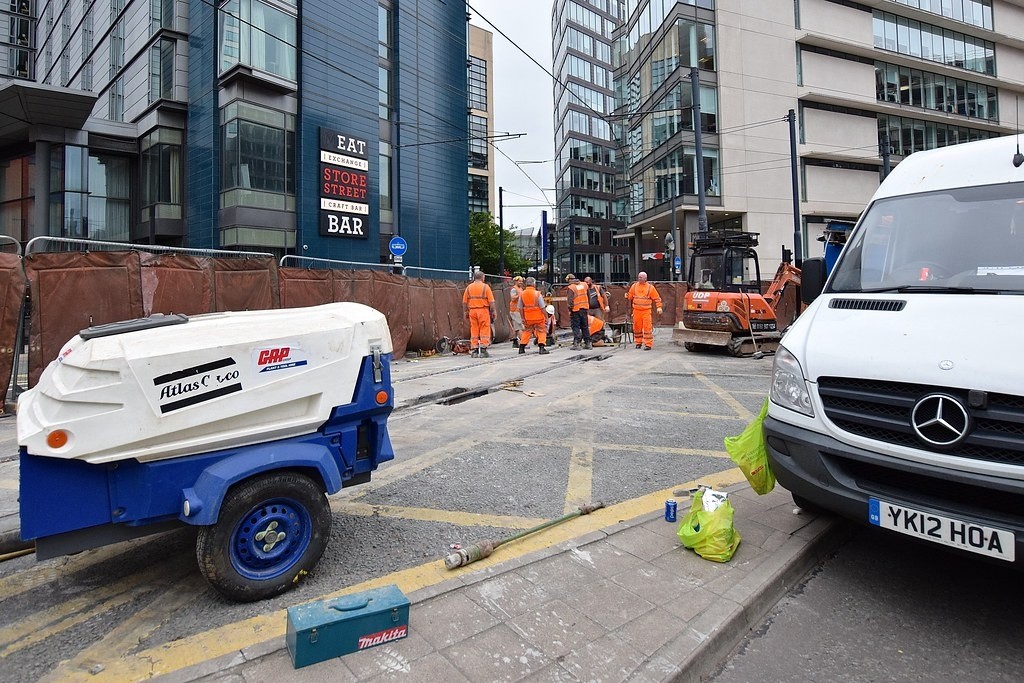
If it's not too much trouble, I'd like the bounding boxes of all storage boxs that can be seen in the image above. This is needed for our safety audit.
[285,583,411,670]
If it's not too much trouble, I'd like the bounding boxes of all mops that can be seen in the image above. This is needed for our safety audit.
[739,288,764,360]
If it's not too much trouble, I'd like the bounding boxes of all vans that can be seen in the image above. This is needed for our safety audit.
[762,134,1023,571]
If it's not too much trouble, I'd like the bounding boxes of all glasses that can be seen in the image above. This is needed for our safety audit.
[548,314,552,315]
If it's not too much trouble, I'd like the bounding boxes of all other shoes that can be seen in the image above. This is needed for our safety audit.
[510,338,519,348]
[471,349,478,358]
[570,345,580,350]
[644,346,651,350]
[538,345,549,354]
[533,338,538,345]
[519,344,526,354]
[583,342,591,349]
[635,344,641,349]
[480,347,489,357]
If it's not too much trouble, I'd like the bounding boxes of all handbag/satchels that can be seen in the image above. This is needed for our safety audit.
[676,488,741,563]
[723,397,777,497]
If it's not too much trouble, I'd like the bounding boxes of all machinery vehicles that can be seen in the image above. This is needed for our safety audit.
[670,229,809,359]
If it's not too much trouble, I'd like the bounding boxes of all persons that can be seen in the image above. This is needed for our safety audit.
[462,271,497,358]
[509,276,530,349]
[625,272,662,350]
[584,277,609,321]
[517,277,549,354]
[565,274,592,350]
[580,315,612,346]
[703,261,724,285]
[533,305,556,347]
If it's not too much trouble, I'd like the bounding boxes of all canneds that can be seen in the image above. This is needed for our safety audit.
[664,499,677,523]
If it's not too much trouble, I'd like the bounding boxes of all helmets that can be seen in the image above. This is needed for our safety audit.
[545,305,555,315]
